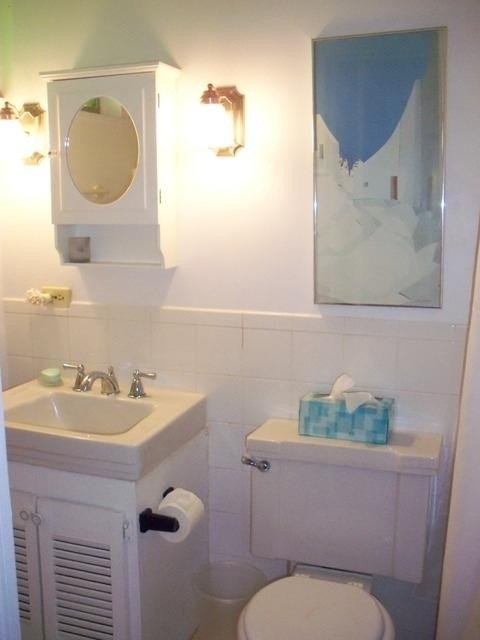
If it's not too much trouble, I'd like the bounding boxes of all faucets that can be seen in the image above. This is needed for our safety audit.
[79,364,119,393]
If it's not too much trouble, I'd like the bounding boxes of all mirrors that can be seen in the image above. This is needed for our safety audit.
[65,94,140,206]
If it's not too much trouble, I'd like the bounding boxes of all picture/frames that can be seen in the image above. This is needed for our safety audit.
[311,25,446,309]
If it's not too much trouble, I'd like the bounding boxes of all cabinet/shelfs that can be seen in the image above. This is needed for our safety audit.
[8,427,210,639]
[39,59,183,226]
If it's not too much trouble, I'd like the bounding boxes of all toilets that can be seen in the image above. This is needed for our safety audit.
[236,418,441,640]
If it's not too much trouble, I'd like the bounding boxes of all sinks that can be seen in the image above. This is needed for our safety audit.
[1,375,208,480]
